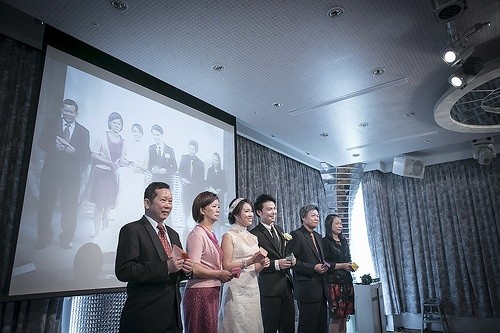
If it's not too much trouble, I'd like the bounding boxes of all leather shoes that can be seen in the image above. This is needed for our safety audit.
[35,232,54,249]
[59,232,73,249]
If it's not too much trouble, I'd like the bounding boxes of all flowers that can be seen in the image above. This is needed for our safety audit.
[282,232,293,243]
[164,153,172,162]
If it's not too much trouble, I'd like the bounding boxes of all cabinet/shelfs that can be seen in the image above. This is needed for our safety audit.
[352,280,386,333]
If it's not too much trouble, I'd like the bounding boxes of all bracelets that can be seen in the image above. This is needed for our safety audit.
[243,258,247,268]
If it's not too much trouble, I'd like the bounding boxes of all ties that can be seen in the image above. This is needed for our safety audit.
[189,160,193,179]
[312,234,319,256]
[270,225,278,242]
[156,225,173,257]
[158,147,161,158]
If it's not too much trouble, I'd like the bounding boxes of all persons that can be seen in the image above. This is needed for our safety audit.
[37,98,228,290]
[290,204,330,333]
[218,198,270,333]
[183,191,233,333]
[250,194,296,333]
[322,214,356,333]
[114,181,194,333]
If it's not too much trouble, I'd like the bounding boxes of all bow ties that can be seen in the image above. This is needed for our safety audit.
[63,124,71,141]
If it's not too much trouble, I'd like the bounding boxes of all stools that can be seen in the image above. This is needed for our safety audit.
[421,298,450,333]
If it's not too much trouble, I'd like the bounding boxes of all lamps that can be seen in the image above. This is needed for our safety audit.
[440,37,484,88]
